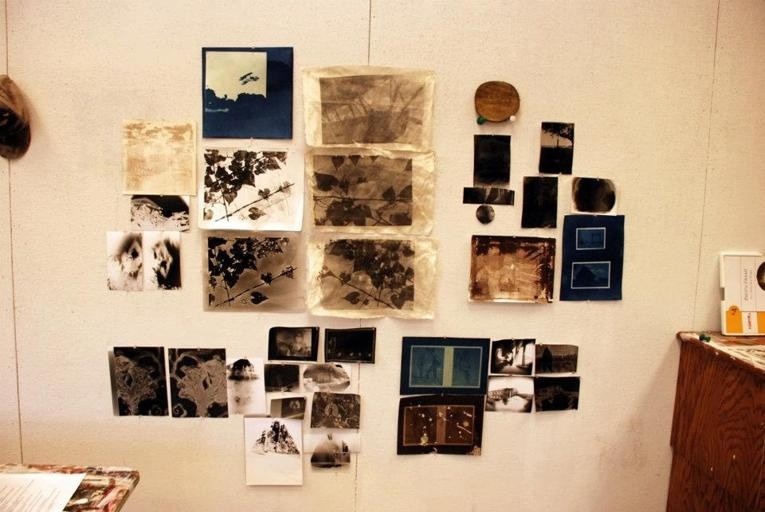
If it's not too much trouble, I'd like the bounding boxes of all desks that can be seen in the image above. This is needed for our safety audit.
[0,461,142,512]
[665,328,764,512]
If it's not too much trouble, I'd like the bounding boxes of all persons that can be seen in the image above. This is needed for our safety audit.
[255,421,290,452]
